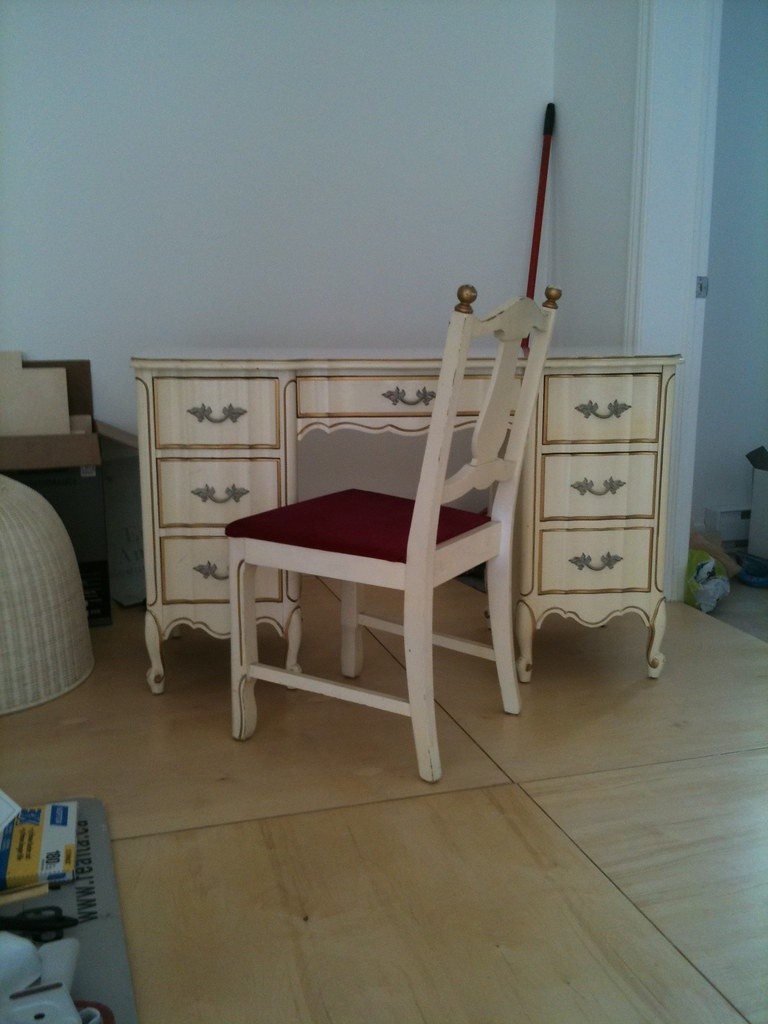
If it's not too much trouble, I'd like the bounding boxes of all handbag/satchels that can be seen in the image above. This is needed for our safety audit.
[685,530,742,614]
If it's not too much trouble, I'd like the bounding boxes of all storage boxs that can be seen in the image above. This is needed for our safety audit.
[0,802,78,891]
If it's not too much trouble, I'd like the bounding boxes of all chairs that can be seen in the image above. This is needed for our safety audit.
[227,285,562,782]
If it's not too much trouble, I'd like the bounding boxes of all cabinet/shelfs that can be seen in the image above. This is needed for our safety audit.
[128,355,686,694]
